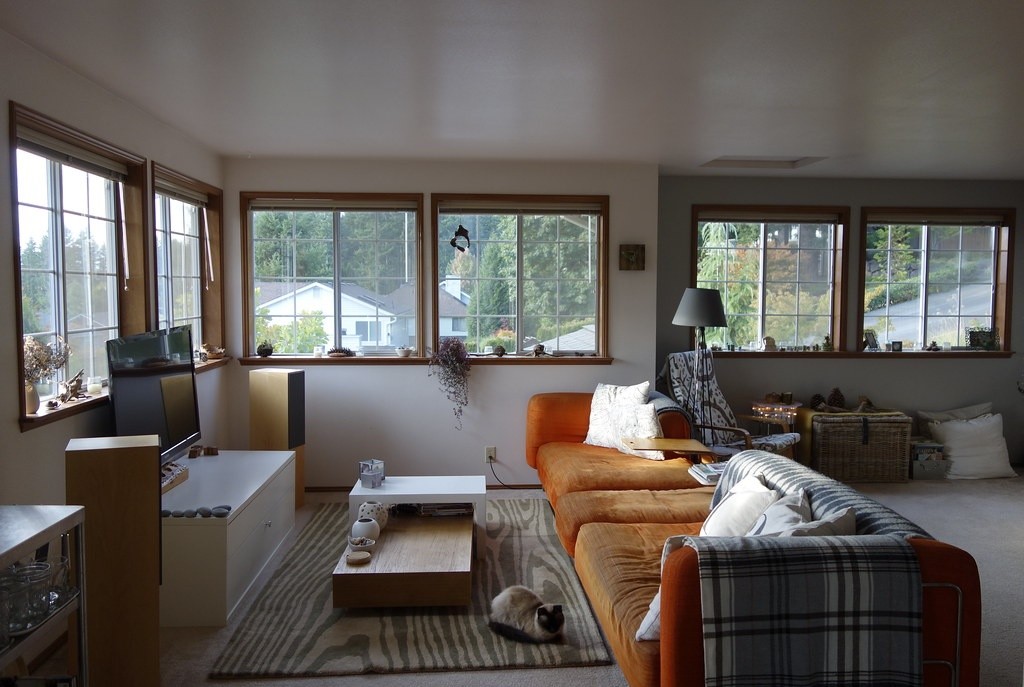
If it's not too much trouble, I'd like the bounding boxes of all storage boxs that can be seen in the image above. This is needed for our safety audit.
[799,411,913,483]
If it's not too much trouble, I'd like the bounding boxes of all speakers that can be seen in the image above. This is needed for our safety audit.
[64,434,163,687]
[248,367,307,512]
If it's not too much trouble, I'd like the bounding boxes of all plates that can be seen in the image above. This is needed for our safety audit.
[45,406,57,409]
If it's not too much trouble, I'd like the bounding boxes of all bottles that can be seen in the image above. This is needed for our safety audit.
[352,518,380,541]
[358,501,388,530]
[728,343,741,351]
[485,346,493,355]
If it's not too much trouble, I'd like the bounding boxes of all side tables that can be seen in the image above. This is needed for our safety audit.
[622,437,710,454]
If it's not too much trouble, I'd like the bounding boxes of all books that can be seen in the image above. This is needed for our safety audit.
[688,461,727,487]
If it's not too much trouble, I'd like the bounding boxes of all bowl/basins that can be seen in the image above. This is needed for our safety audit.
[395,349,412,357]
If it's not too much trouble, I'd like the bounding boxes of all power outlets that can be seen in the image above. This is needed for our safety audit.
[486,445,496,463]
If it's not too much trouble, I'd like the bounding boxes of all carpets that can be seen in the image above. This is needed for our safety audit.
[211,497,610,677]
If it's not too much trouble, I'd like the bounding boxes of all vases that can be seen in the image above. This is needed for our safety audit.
[25,384,42,416]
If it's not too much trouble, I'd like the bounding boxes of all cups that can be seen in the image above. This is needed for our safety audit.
[750,342,755,351]
[32,557,70,606]
[10,562,51,618]
[886,342,892,351]
[87,376,102,394]
[193,353,200,364]
[356,347,364,356]
[943,342,951,351]
[0,576,30,649]
[914,342,922,351]
[712,342,719,350]
[314,347,322,357]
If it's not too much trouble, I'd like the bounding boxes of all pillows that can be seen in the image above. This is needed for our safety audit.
[583,381,651,446]
[749,487,813,536]
[699,474,776,541]
[637,592,663,643]
[917,400,992,440]
[608,406,667,460]
[928,413,1018,481]
[781,508,859,538]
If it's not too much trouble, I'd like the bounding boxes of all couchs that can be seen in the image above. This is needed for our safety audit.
[526,393,982,687]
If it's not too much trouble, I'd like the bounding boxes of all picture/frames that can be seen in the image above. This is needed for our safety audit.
[863,329,880,352]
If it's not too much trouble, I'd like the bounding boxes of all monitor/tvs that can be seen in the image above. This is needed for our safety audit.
[105,324,202,469]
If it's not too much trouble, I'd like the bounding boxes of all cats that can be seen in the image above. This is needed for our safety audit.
[489,585,565,643]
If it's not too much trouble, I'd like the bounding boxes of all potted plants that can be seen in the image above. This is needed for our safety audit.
[256,339,274,357]
[966,327,1002,350]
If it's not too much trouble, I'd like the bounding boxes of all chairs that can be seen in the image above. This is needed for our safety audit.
[656,350,801,463]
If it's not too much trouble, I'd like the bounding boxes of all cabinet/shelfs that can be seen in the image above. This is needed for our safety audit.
[163,448,298,629]
[0,507,90,687]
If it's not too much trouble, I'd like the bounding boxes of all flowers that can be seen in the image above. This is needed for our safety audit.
[426,338,471,430]
[26,334,70,383]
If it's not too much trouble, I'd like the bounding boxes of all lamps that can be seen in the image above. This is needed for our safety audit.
[673,285,725,433]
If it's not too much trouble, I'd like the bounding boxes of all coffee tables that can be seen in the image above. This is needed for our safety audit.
[331,474,488,610]
